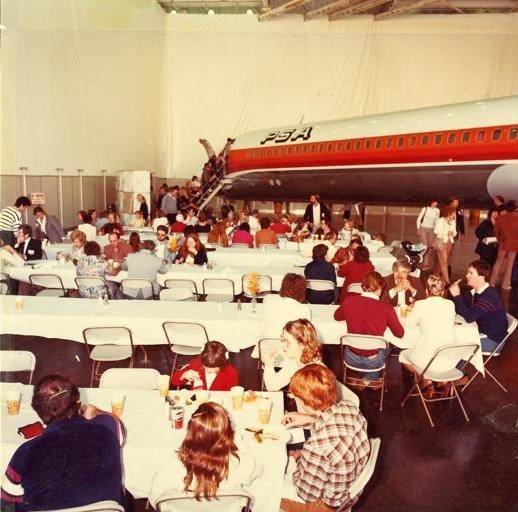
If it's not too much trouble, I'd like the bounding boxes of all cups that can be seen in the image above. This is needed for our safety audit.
[5,390,22,416]
[230,386,244,410]
[15,296,24,310]
[156,375,169,398]
[400,304,408,319]
[111,394,126,417]
[258,400,274,425]
[169,406,185,430]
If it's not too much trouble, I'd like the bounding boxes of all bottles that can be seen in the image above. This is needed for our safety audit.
[98,294,109,305]
[203,262,212,273]
[218,300,224,311]
[236,299,242,312]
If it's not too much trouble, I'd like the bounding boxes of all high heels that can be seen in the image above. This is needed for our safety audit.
[418,385,451,399]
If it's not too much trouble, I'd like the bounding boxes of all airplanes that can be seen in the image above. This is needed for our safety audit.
[195,93,518,214]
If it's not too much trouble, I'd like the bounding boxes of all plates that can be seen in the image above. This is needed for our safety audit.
[245,425,292,448]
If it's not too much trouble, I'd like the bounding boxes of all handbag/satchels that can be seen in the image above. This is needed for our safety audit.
[432,237,444,251]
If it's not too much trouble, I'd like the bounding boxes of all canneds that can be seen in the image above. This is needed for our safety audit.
[170,406,184,430]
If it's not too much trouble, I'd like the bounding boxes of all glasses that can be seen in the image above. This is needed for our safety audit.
[280,338,301,344]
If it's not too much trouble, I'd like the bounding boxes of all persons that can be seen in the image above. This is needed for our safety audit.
[263,316,329,416]
[144,400,263,512]
[250,272,314,359]
[169,339,240,391]
[332,270,405,391]
[398,274,457,399]
[280,363,372,512]
[449,258,509,388]
[0,373,129,512]
[1,154,517,304]
